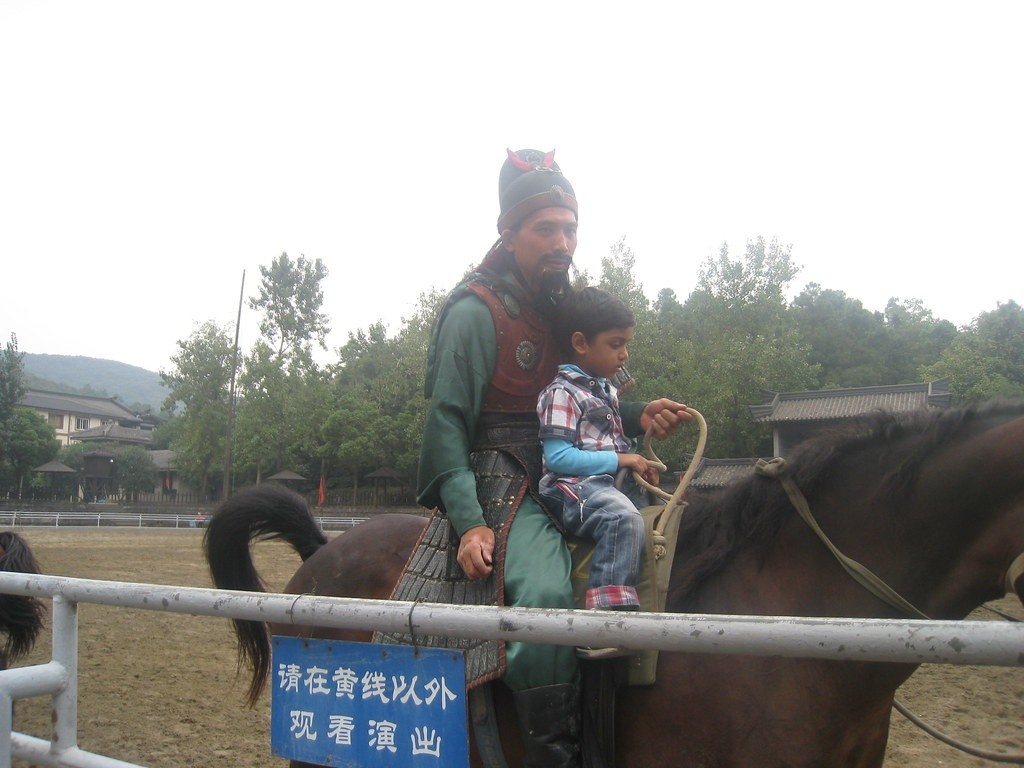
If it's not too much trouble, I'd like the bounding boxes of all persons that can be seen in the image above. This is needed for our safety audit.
[195,512,204,528]
[535,286,650,660]
[415,148,694,768]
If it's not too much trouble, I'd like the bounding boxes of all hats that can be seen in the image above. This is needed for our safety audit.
[497,147,578,234]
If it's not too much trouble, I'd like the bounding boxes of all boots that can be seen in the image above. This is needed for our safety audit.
[512,679,582,768]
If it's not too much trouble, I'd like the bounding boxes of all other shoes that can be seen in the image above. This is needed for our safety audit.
[575,643,641,660]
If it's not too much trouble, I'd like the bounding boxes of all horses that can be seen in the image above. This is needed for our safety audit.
[0,530,48,673]
[201,399,1024,768]
